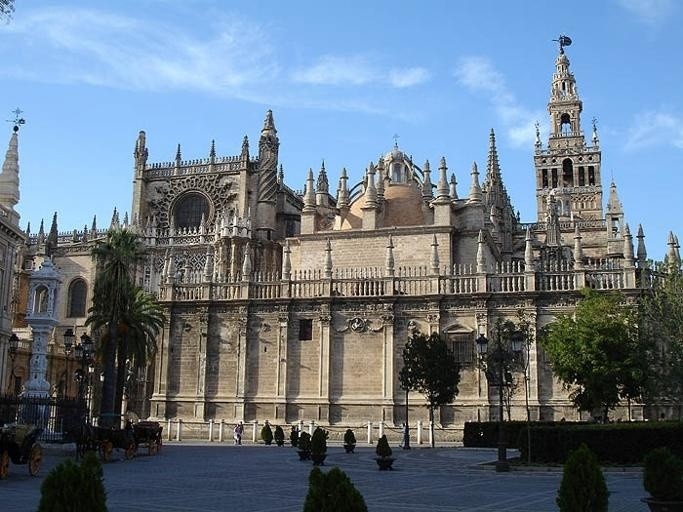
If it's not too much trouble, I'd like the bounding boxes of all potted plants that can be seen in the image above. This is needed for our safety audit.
[260,425,329,466]
[373,435,397,471]
[343,429,358,452]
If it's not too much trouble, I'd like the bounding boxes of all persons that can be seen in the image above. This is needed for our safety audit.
[399,423,406,448]
[658,413,667,421]
[592,415,609,424]
[233,421,244,445]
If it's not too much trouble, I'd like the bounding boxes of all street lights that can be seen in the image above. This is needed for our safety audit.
[8,332,18,396]
[474,328,524,473]
[63,328,95,402]
